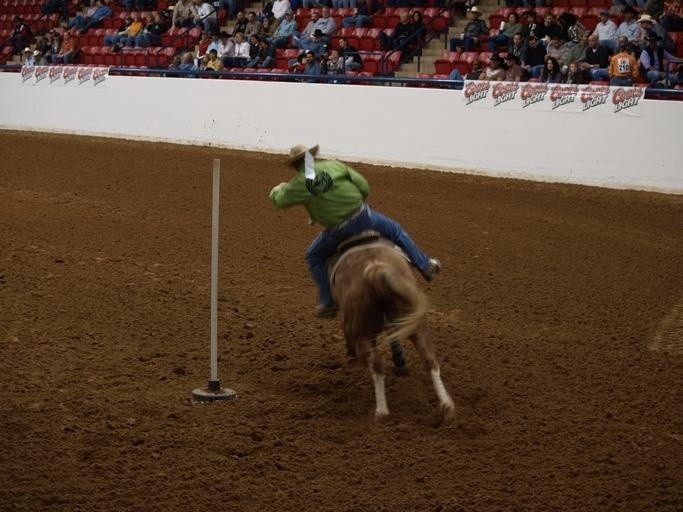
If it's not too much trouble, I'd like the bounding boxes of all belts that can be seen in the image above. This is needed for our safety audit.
[329,204,365,231]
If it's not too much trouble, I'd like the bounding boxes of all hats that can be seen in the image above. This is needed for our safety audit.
[23,47,32,52]
[636,15,656,23]
[219,31,232,38]
[312,29,325,36]
[621,6,638,14]
[33,50,40,56]
[600,11,610,16]
[505,54,515,59]
[470,6,481,12]
[489,54,503,62]
[284,144,319,166]
[285,9,293,15]
[644,31,662,40]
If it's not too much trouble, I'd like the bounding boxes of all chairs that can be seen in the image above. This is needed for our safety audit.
[219,9,227,19]
[387,15,401,27]
[88,64,96,67]
[358,72,378,84]
[7,14,15,21]
[609,14,620,27]
[83,46,91,54]
[409,73,433,86]
[230,68,243,78]
[370,51,386,60]
[141,11,152,19]
[119,11,129,20]
[346,36,360,50]
[32,14,40,20]
[280,49,298,58]
[588,80,609,86]
[17,14,23,18]
[104,53,115,64]
[25,4,33,13]
[94,52,104,64]
[385,50,401,69]
[424,8,440,17]
[130,11,140,20]
[9,0,17,6]
[98,64,107,67]
[275,57,288,69]
[459,52,478,68]
[395,8,410,15]
[496,7,514,19]
[199,44,208,56]
[135,53,146,65]
[432,74,448,86]
[515,7,532,16]
[633,84,649,87]
[114,11,120,19]
[101,47,111,53]
[40,13,47,21]
[423,14,431,25]
[367,28,381,38]
[257,68,271,80]
[378,57,393,73]
[534,6,550,17]
[337,8,354,16]
[409,8,425,16]
[88,35,98,45]
[77,64,88,67]
[88,28,95,35]
[375,36,380,49]
[383,28,393,35]
[50,13,58,22]
[24,21,32,26]
[552,6,570,17]
[164,46,176,56]
[434,59,451,74]
[385,8,394,16]
[133,47,144,54]
[360,35,373,51]
[331,35,341,48]
[451,60,469,75]
[432,15,448,31]
[332,15,343,28]
[127,65,138,76]
[72,35,79,46]
[29,24,37,33]
[177,27,188,37]
[145,47,155,55]
[352,28,368,37]
[442,8,454,18]
[363,57,378,73]
[12,22,20,28]
[244,68,257,79]
[581,14,598,31]
[276,48,284,59]
[16,5,25,13]
[2,45,13,54]
[104,18,113,28]
[478,52,492,64]
[95,29,105,36]
[162,35,172,46]
[0,0,8,6]
[37,0,45,6]
[271,69,284,80]
[104,28,112,35]
[190,27,202,38]
[608,5,625,21]
[28,0,36,6]
[114,18,123,28]
[172,36,184,48]
[223,68,230,79]
[152,11,158,16]
[123,46,133,54]
[587,6,606,17]
[78,35,89,46]
[18,0,27,6]
[528,78,538,82]
[114,53,123,65]
[0,21,4,30]
[358,50,370,61]
[8,6,16,14]
[328,8,336,16]
[309,8,322,15]
[99,35,104,46]
[85,53,94,64]
[146,54,157,68]
[488,14,506,29]
[169,28,178,36]
[4,21,12,29]
[0,6,7,13]
[139,65,148,76]
[297,8,309,15]
[0,14,6,21]
[90,47,101,54]
[158,54,169,67]
[24,14,31,20]
[154,46,166,54]
[109,65,118,75]
[45,20,53,29]
[124,53,135,66]
[36,21,44,29]
[339,26,353,37]
[497,53,509,58]
[345,71,357,83]
[373,14,386,28]
[2,29,13,37]
[33,4,41,13]
[569,6,589,19]
[441,52,458,62]
[118,65,128,77]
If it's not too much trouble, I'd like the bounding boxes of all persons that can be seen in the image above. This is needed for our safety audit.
[451,60,485,81]
[450,7,487,54]
[480,0,682,97]
[269,144,440,319]
[8,0,174,66]
[160,1,389,88]
[376,10,429,59]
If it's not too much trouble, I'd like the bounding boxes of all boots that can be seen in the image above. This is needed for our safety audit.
[421,258,440,283]
[313,302,337,317]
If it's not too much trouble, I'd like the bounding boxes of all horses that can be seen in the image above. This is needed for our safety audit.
[326,229,458,426]
